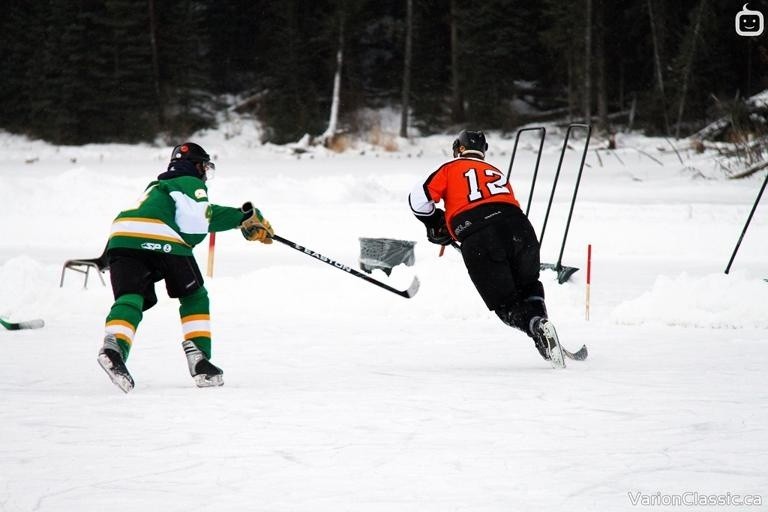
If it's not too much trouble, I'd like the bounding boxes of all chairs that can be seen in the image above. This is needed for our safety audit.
[60,238,112,288]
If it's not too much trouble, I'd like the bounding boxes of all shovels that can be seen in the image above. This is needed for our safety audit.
[539,123,593,283]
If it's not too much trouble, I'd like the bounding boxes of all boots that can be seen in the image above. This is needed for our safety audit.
[182,340,223,380]
[527,300,560,362]
[99,334,135,390]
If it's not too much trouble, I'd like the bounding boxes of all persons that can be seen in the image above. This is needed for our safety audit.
[99,143,274,389]
[408,129,563,365]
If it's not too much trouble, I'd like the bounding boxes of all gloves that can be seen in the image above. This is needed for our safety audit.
[240,202,274,244]
[426,208,454,246]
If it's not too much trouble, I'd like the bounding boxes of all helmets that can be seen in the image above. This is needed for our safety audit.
[171,143,215,179]
[453,129,488,158]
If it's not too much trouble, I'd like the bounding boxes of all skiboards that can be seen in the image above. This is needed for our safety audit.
[450,242,588,360]
[269,235,420,298]
[0,319,45,331]
